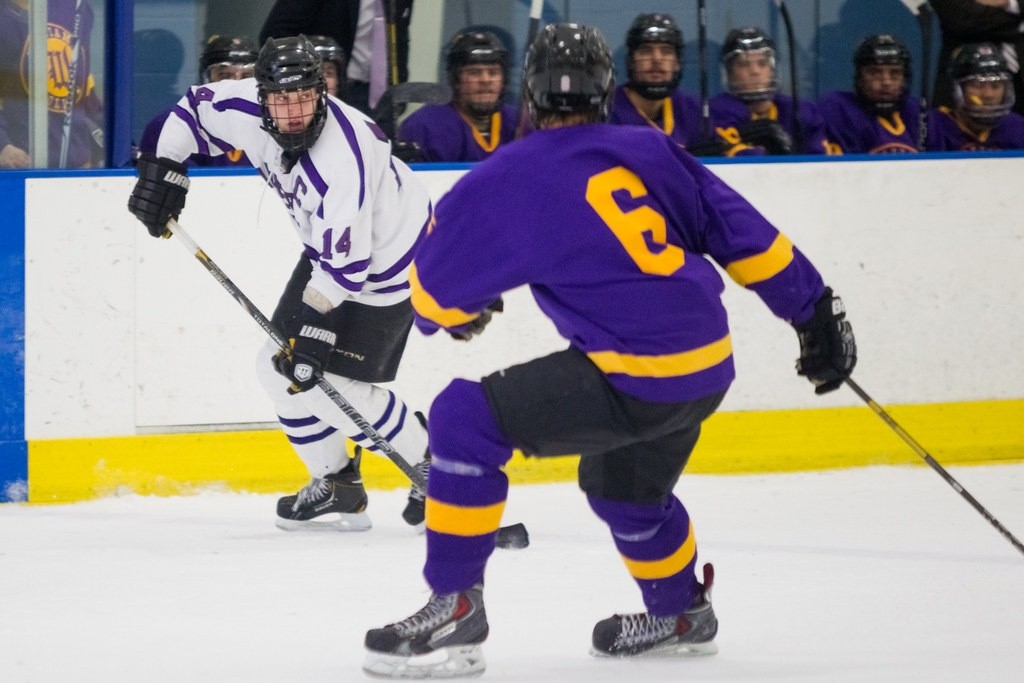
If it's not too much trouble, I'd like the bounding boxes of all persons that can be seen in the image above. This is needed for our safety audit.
[0,0,105,169]
[361,22,858,679]
[131,32,437,536]
[126,0,1024,170]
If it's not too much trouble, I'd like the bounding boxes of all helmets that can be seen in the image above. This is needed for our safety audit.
[522,21,614,123]
[940,43,1015,128]
[719,27,781,100]
[852,35,912,116]
[445,29,509,116]
[308,36,346,97]
[253,34,328,152]
[200,34,259,85]
[625,12,684,100]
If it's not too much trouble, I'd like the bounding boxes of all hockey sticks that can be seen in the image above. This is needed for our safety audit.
[697,0,712,141]
[58,0,85,170]
[841,372,1024,554]
[515,0,544,135]
[388,0,401,153]
[775,1,804,154]
[164,217,529,550]
[901,0,934,152]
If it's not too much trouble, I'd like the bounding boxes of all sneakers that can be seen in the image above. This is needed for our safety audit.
[362,576,489,679]
[274,446,371,531]
[402,411,432,534]
[588,562,718,659]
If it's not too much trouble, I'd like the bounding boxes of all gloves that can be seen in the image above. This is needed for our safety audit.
[451,298,504,342]
[794,287,858,396]
[127,153,190,239]
[737,118,800,156]
[687,137,728,158]
[271,302,343,392]
